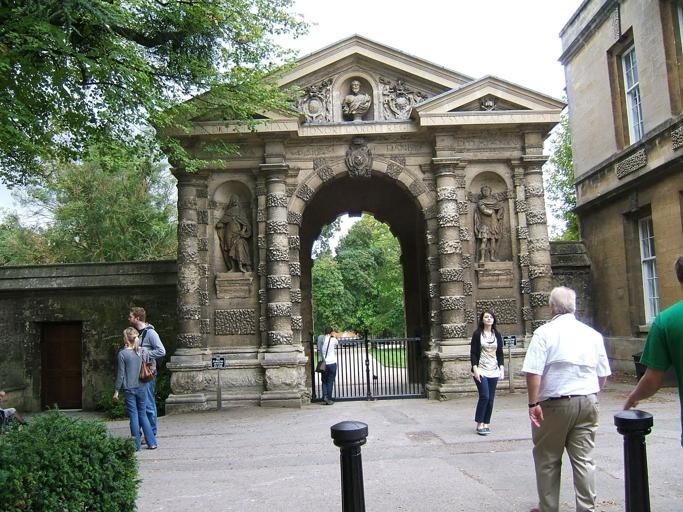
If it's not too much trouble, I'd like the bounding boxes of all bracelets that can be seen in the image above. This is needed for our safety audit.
[528,402,539,407]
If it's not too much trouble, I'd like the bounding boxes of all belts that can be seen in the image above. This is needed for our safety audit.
[548,395,582,400]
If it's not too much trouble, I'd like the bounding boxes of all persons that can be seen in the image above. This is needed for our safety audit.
[0,391,27,427]
[215,192,251,274]
[383,82,426,117]
[519,283,614,511]
[112,327,158,452]
[469,309,505,435]
[620,255,683,450]
[316,327,339,406]
[127,307,167,444]
[342,80,371,120]
[476,183,504,264]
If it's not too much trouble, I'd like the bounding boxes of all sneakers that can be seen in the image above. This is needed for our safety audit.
[476,426,491,435]
[322,396,335,406]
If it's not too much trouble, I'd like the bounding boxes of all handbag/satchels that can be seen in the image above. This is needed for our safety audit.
[314,359,326,372]
[138,361,156,382]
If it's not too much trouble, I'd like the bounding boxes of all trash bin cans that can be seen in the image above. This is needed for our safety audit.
[632,352,647,382]
